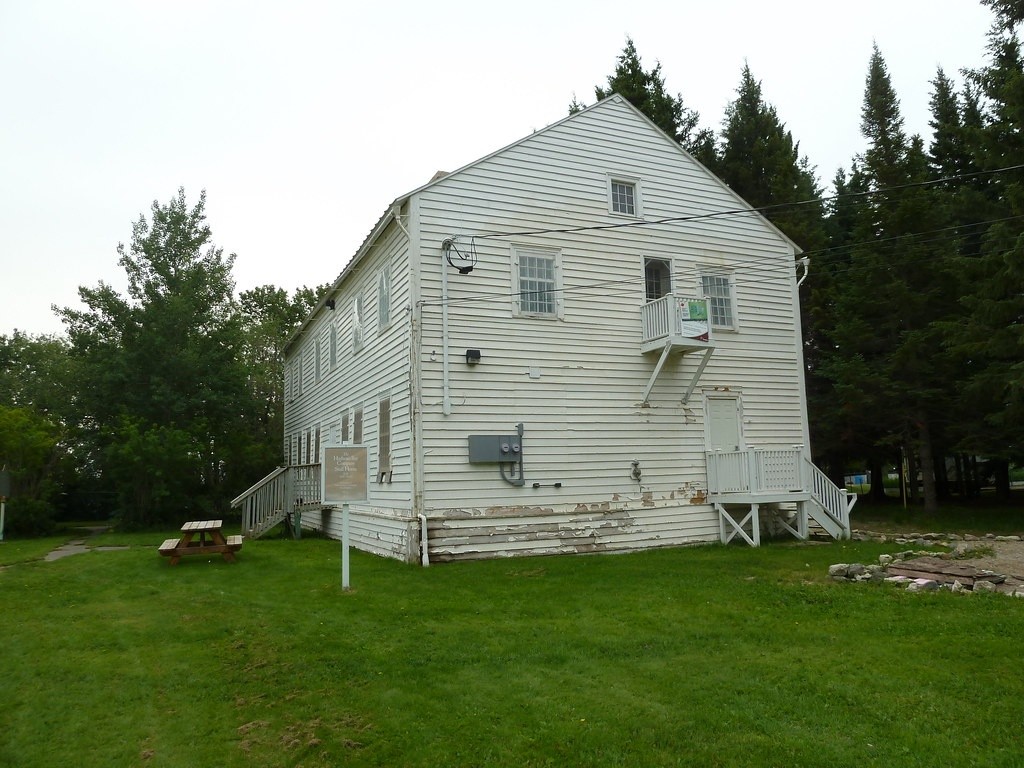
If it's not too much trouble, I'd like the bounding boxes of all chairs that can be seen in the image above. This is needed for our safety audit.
[226,534,242,552]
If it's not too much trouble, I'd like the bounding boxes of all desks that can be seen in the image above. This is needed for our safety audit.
[170,520,236,567]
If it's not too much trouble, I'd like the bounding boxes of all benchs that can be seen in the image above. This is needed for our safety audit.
[159,539,181,555]
